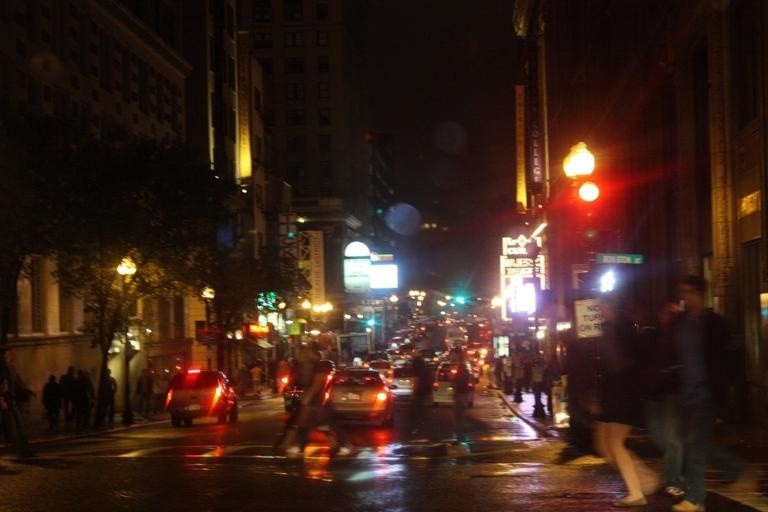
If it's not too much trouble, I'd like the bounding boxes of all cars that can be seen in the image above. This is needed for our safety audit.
[276,312,493,420]
[167,369,238,427]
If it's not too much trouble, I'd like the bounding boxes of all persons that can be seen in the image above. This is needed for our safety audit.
[494,350,562,418]
[595,318,662,510]
[654,277,733,512]
[404,344,476,448]
[42,366,117,430]
[251,341,369,456]
[133,369,172,417]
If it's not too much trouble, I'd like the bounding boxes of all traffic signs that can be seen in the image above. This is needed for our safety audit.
[596,252,644,264]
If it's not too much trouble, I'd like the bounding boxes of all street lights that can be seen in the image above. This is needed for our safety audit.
[114,255,139,426]
[524,236,542,331]
[201,283,217,370]
[560,140,597,255]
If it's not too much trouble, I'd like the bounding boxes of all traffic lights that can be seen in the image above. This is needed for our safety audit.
[578,180,603,247]
